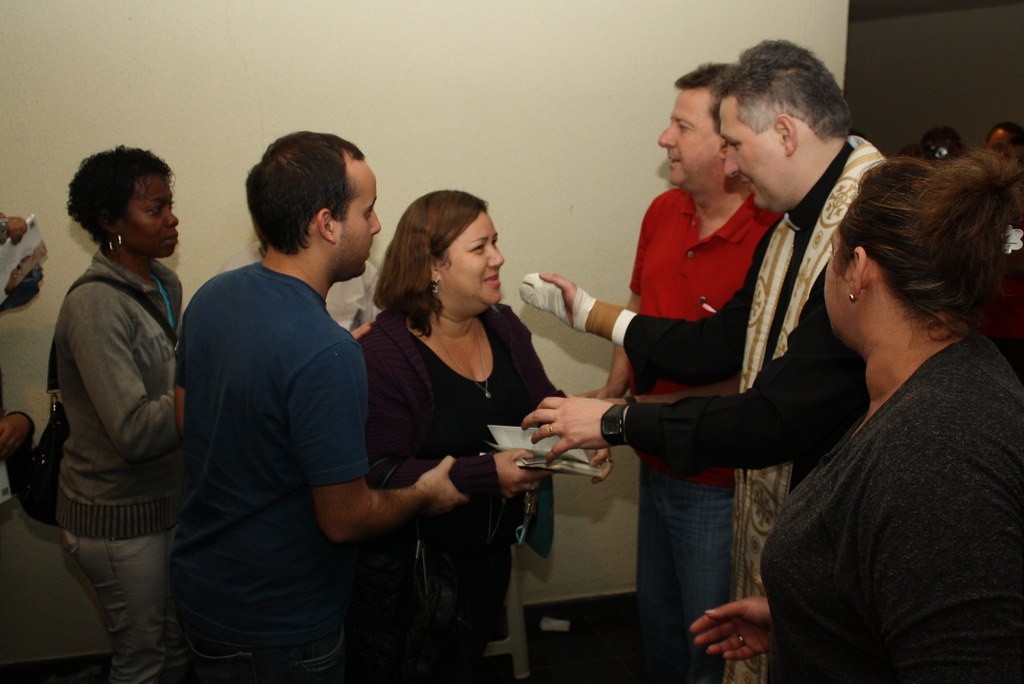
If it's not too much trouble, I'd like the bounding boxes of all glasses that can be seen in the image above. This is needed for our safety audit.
[921,142,952,162]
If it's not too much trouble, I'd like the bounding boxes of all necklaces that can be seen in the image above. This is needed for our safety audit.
[433,320,491,398]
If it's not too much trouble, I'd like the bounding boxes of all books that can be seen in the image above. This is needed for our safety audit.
[0,215,47,306]
[480,424,603,477]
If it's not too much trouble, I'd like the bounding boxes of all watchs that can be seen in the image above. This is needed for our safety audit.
[601,403,627,446]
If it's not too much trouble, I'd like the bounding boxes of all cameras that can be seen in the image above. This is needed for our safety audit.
[921,143,951,160]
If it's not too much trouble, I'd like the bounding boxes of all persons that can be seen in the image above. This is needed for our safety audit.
[355,190,614,684]
[54,147,186,684]
[689,158,1024,684]
[176,131,469,684]
[576,64,784,684]
[521,39,888,684]
[924,122,1024,382]
[0,212,43,491]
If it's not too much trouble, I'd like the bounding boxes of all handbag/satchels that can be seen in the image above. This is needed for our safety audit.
[19,401,69,526]
[345,457,472,684]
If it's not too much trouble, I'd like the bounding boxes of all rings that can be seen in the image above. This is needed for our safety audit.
[738,633,745,646]
[548,422,553,436]
